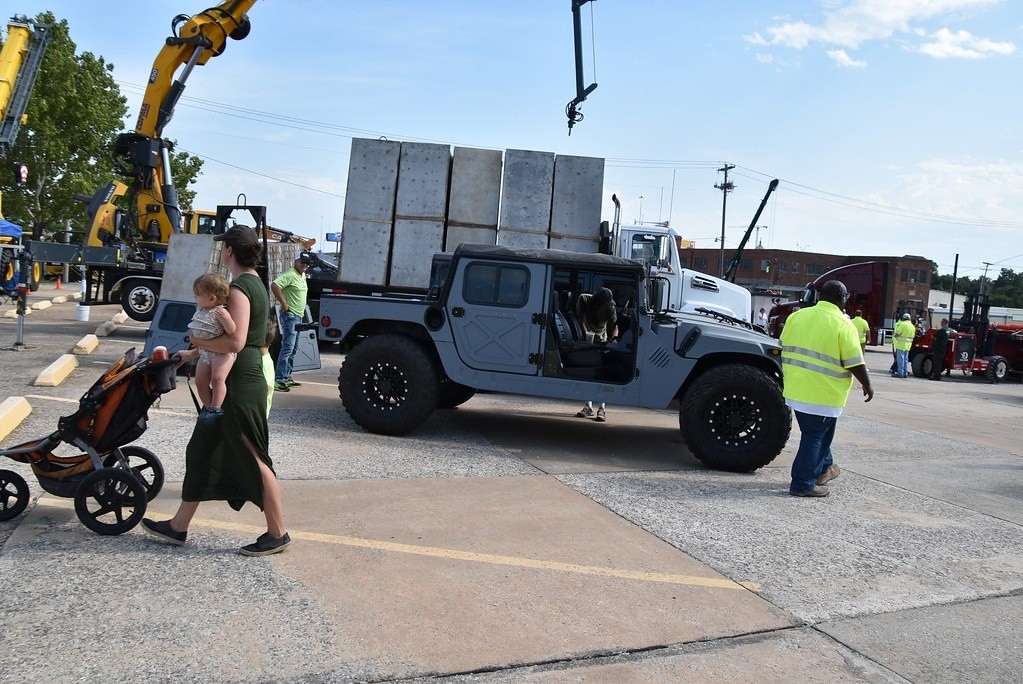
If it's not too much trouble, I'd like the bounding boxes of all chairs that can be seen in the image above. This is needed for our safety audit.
[551,290,615,381]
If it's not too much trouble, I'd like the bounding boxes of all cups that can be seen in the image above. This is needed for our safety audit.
[586,332,595,344]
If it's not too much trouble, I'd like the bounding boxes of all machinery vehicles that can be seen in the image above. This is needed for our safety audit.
[0,13,57,301]
[0,0,598,351]
[723,179,779,284]
[908,293,1023,384]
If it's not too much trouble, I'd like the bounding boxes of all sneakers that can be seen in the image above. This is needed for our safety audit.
[789,463,840,498]
[239,532,292,556]
[141,518,188,545]
[274,378,301,392]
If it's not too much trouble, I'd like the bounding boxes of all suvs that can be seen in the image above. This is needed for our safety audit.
[319,244,793,475]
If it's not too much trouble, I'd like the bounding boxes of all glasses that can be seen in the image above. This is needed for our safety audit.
[300,261,309,265]
[839,291,850,299]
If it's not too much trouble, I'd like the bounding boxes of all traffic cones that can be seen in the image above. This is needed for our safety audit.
[54,279,62,290]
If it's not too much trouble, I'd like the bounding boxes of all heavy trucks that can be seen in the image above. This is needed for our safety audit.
[319,194,751,329]
[767,254,934,345]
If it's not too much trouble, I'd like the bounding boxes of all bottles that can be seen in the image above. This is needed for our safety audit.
[153,346,169,363]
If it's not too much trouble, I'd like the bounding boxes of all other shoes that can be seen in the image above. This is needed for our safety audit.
[929,376,942,381]
[596,408,607,421]
[576,405,595,417]
[196,406,223,424]
[892,374,908,378]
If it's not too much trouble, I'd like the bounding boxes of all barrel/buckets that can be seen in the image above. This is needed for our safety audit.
[76,305,90,321]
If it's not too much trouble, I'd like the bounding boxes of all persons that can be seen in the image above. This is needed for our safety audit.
[140,225,292,556]
[889,312,915,378]
[757,307,767,328]
[188,273,238,419]
[851,310,871,372]
[930,318,950,380]
[271,252,309,393]
[779,280,874,496]
[575,287,618,421]
[260,319,275,419]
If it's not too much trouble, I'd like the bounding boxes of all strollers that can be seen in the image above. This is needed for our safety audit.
[0,347,181,536]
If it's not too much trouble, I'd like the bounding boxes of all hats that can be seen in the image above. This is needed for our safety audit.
[902,312,911,320]
[591,287,617,306]
[295,251,310,260]
[213,225,259,246]
